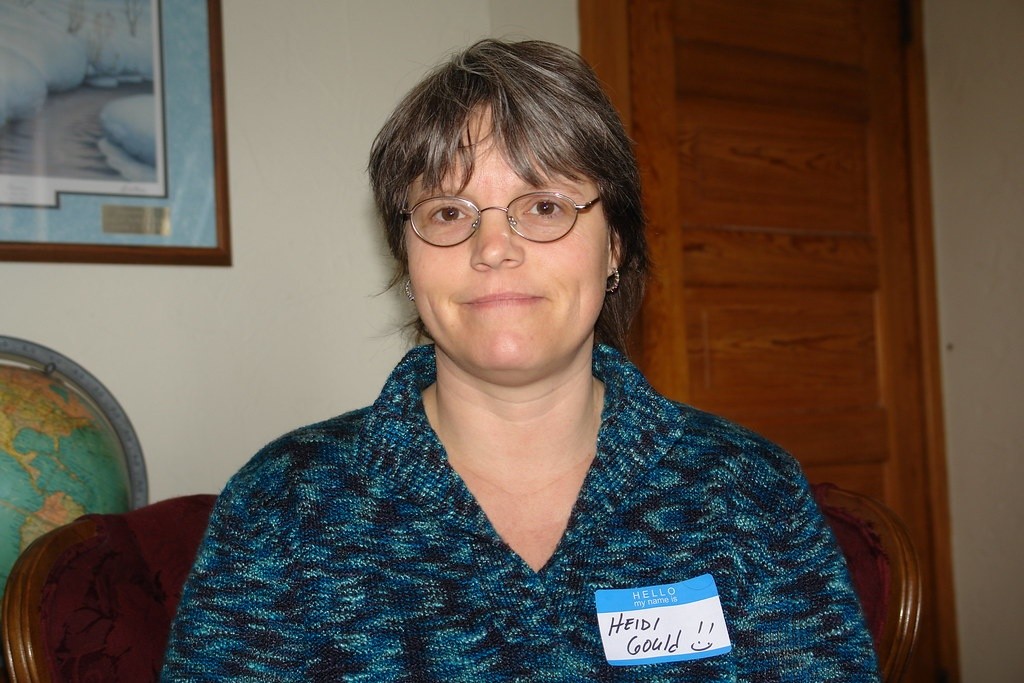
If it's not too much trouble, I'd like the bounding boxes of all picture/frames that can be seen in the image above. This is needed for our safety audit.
[0,0,232,268]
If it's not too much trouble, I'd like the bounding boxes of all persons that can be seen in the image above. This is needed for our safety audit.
[160,37,879,683]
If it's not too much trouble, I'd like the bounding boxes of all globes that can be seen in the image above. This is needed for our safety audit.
[1,334,150,608]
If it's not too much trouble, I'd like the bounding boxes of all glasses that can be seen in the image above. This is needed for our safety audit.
[399,182,602,248]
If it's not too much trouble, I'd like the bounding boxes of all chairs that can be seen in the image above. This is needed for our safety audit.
[0,480,925,683]
